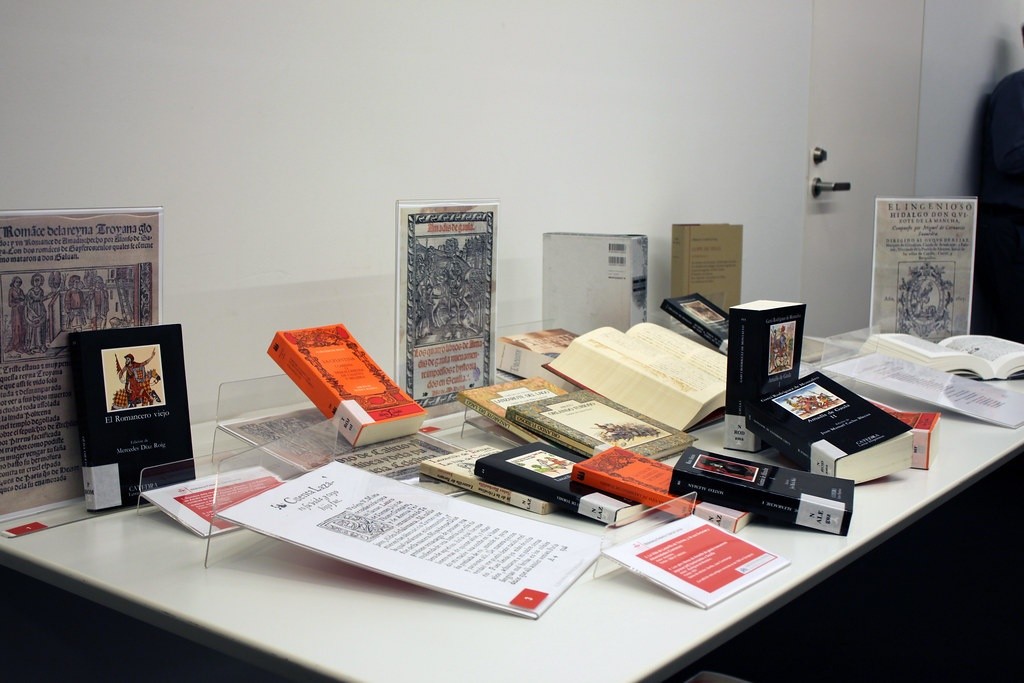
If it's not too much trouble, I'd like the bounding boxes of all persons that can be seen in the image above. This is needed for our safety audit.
[972,26,1024,345]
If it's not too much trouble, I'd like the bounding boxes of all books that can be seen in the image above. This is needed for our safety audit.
[64,324,196,513]
[868,195,978,343]
[422,293,1024,538]
[670,222,742,314]
[268,322,426,447]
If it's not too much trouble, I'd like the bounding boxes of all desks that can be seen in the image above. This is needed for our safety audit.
[2,324,1024,683]
